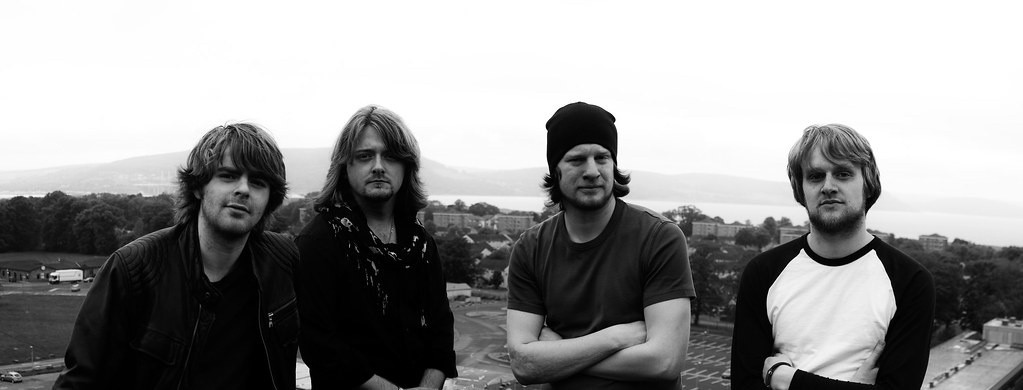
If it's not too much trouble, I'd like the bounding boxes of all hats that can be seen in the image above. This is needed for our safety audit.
[545,101,618,171]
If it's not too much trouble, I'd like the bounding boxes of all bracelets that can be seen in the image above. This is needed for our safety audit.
[764,361,791,389]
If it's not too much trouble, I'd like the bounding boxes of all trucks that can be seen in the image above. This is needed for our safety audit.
[48,269,83,284]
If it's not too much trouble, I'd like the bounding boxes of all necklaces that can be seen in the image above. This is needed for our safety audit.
[370,213,397,244]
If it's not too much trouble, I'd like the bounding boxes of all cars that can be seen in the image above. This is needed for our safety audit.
[71,284,80,291]
[84,277,93,283]
[721,368,731,379]
[1,372,23,383]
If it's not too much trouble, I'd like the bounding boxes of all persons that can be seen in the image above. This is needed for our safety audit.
[292,105,460,390]
[1,271,29,282]
[731,123,932,390]
[506,100,697,389]
[56,120,304,390]
